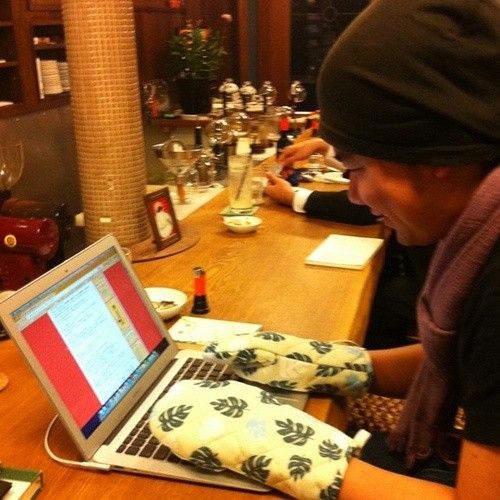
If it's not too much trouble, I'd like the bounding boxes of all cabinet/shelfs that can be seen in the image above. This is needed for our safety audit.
[256,0,370,110]
[0,0,71,118]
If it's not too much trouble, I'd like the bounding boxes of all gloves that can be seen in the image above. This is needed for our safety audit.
[148,379,373,500]
[202,331,374,400]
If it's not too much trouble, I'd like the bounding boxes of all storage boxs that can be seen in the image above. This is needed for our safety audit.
[0,466,43,500]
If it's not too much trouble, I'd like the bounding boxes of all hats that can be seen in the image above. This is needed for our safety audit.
[318,0,500,168]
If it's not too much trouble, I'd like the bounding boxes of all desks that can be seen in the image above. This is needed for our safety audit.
[0,153,394,500]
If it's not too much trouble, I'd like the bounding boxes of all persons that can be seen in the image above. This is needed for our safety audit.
[263,136,437,347]
[149,1,500,499]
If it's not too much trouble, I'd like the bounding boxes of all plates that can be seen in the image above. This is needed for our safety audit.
[40,58,71,95]
[323,171,350,184]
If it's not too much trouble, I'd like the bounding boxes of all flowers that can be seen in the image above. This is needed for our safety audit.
[164,12,234,80]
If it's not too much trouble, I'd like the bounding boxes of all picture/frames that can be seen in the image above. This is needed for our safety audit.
[143,188,182,250]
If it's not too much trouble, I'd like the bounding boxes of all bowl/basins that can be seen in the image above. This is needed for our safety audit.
[223,216,262,233]
[144,286,187,319]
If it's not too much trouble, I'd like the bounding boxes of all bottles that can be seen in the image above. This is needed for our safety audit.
[276,118,291,159]
[311,120,319,137]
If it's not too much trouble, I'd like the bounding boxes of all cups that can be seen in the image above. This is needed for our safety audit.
[227,154,254,213]
[121,248,132,263]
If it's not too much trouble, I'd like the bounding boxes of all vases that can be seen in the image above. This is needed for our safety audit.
[178,77,212,114]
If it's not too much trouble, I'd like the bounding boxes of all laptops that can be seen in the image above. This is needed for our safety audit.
[0,234,309,494]
